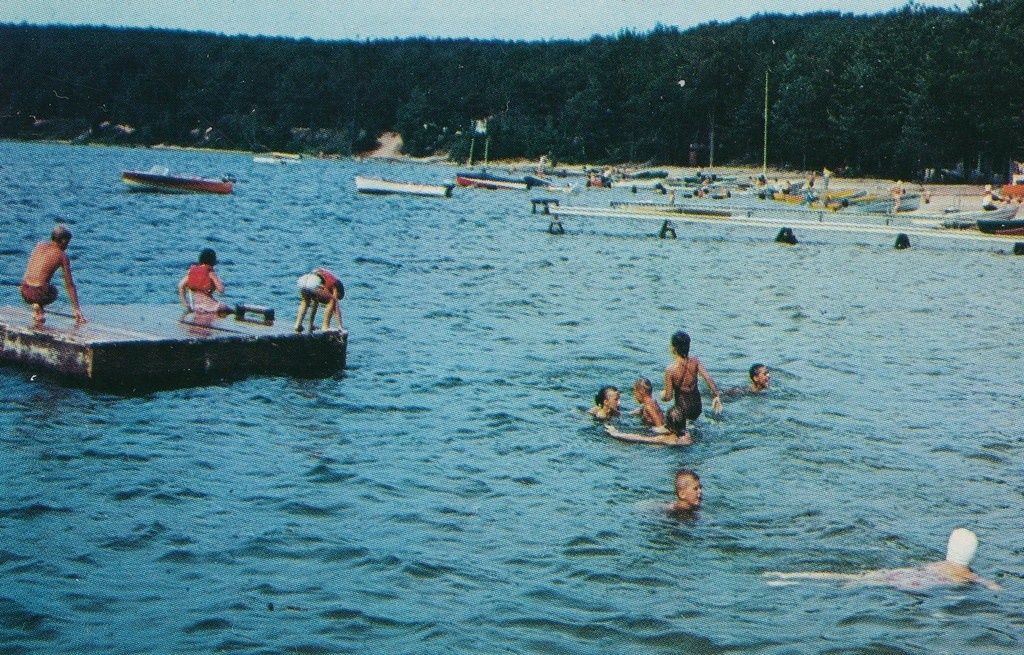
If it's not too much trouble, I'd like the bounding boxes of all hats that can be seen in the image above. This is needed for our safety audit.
[946,526,979,569]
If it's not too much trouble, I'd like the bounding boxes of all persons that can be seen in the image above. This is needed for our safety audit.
[947,528,978,565]
[294,269,344,331]
[983,184,1003,211]
[21,227,86,324]
[589,331,721,444]
[749,363,768,388]
[675,471,702,506]
[179,248,227,312]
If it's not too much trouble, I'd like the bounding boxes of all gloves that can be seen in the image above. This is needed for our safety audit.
[660,389,668,402]
[712,396,722,415]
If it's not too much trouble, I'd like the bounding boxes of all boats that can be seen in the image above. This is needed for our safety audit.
[976,218,1023,235]
[354,173,457,198]
[535,168,923,214]
[454,170,531,190]
[253,155,300,166]
[613,201,730,214]
[121,165,236,196]
[941,206,1019,228]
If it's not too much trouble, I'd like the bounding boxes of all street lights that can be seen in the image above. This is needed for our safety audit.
[678,80,714,167]
